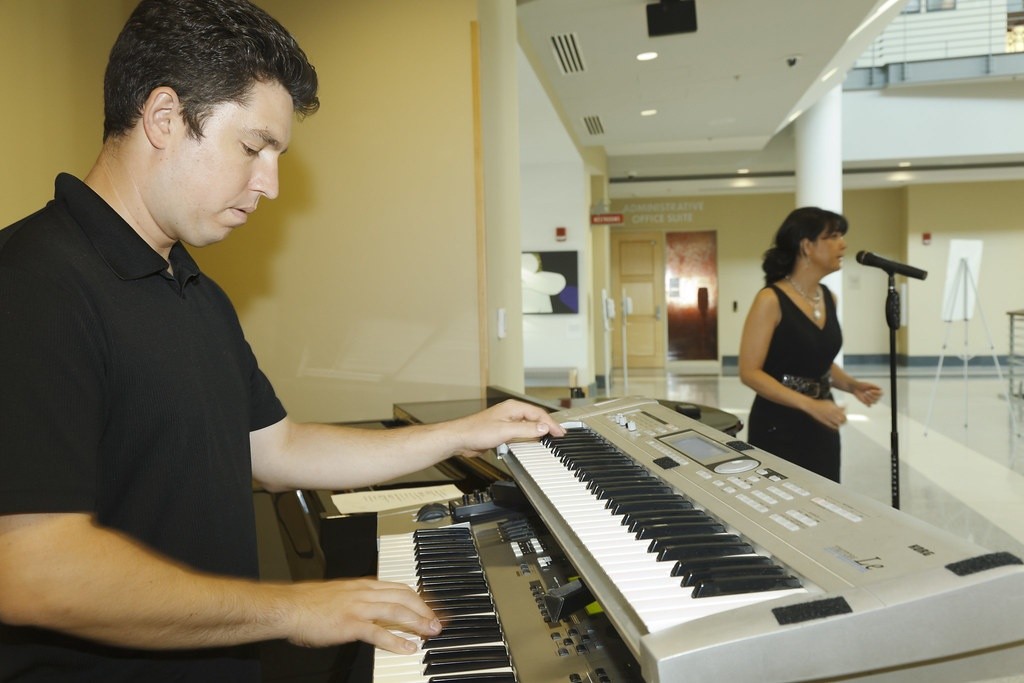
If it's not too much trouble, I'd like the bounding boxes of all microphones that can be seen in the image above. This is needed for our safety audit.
[856,251,927,279]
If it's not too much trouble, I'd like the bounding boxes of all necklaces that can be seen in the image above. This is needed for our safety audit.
[785,275,824,320]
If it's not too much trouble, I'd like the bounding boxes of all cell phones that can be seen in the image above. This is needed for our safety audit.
[450,502,506,525]
[676,404,700,416]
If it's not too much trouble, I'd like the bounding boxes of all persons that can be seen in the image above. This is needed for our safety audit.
[0,0,567,683]
[739,206,884,484]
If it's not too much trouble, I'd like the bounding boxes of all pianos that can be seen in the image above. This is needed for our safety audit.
[368,481,647,683]
[500,395,1024,683]
